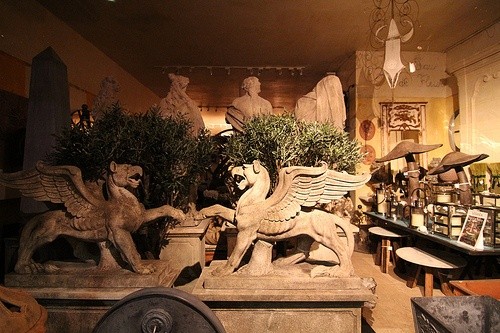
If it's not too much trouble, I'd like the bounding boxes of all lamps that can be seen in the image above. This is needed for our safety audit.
[368,0,419,90]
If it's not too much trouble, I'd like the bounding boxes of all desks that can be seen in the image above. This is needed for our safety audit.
[395,245,468,297]
[362,211,500,280]
[367,226,411,274]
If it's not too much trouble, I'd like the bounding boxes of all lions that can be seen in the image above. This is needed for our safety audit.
[193,159,360,277]
[12,160,187,275]
